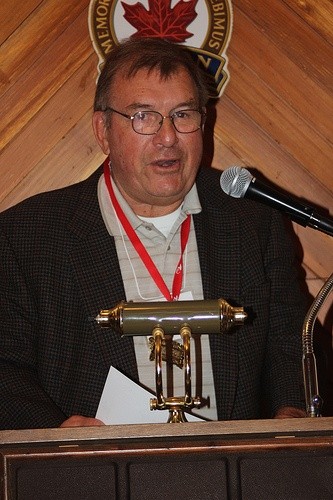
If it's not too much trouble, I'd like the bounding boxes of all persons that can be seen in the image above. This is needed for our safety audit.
[0,40,321,430]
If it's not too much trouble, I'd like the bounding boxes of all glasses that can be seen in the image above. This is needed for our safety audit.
[103,106,208,136]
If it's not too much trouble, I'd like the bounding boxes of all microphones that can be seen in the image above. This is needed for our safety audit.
[218,167,333,236]
